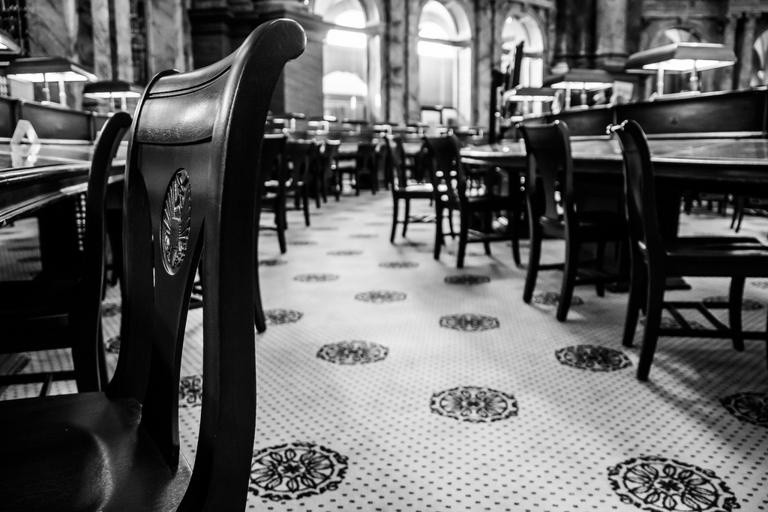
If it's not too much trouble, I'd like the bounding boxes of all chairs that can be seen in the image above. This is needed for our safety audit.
[2,17,306,511]
[423,119,767,321]
[612,121,768,383]
[253,106,465,255]
